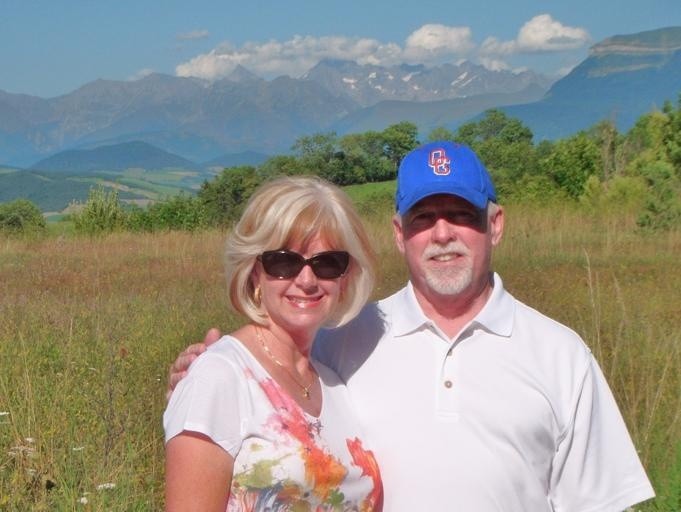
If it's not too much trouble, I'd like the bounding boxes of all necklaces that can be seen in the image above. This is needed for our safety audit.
[253,320,314,403]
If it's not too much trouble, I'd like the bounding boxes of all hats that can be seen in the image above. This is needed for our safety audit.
[395,139,497,217]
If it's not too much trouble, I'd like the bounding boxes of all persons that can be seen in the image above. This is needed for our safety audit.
[164,139,656,512]
[163,174,384,512]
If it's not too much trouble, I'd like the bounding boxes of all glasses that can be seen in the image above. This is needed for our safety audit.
[257,249,350,280]
